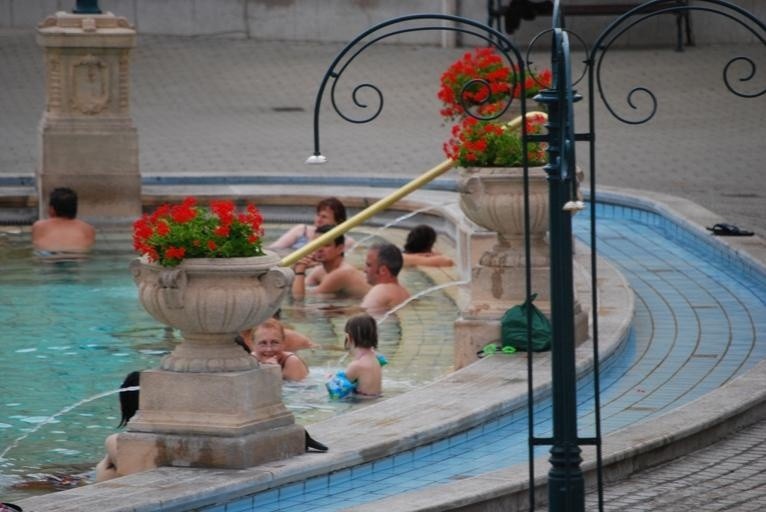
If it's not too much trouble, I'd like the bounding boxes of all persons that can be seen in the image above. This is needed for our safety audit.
[31,187,95,253]
[316,242,410,316]
[93,370,139,482]
[291,224,373,303]
[266,197,356,247]
[401,224,456,269]
[318,315,383,404]
[251,318,309,383]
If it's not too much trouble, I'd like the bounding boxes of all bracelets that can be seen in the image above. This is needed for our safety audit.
[295,272,304,275]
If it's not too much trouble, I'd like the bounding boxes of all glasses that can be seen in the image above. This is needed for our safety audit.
[476,345,517,357]
[255,342,286,352]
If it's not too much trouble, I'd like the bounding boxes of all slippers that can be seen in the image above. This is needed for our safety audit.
[708,223,756,236]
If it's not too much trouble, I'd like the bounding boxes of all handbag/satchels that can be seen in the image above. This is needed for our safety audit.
[502,293,554,351]
